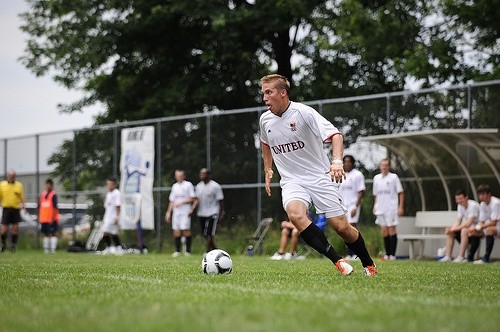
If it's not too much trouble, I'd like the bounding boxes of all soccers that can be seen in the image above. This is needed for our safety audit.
[202,249,233,274]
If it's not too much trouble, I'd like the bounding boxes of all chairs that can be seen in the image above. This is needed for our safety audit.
[238,218,274,254]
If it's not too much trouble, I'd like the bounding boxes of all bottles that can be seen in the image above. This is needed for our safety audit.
[438,248,446,260]
[248,244,254,256]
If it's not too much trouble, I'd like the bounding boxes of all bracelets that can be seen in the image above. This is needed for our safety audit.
[332,159,342,165]
[353,206,357,210]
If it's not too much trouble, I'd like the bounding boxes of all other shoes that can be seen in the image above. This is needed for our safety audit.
[269,252,283,260]
[379,255,396,261]
[43,248,56,254]
[171,251,181,257]
[462,259,471,263]
[344,255,360,261]
[284,253,294,260]
[437,256,451,262]
[0,245,6,253]
[452,256,465,262]
[473,259,486,264]
[10,246,16,253]
[184,252,192,256]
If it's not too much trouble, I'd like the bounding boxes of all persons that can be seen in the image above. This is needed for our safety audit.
[341,155,366,261]
[372,159,404,262]
[102,177,122,255]
[267,211,318,261]
[37,178,60,253]
[0,170,24,252]
[165,167,195,257]
[461,185,500,264]
[438,190,480,263]
[188,168,225,258]
[259,74,378,277]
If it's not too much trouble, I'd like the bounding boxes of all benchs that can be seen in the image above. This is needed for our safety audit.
[398,210,480,260]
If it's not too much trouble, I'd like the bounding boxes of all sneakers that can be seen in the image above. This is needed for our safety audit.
[335,256,354,277]
[364,262,378,277]
